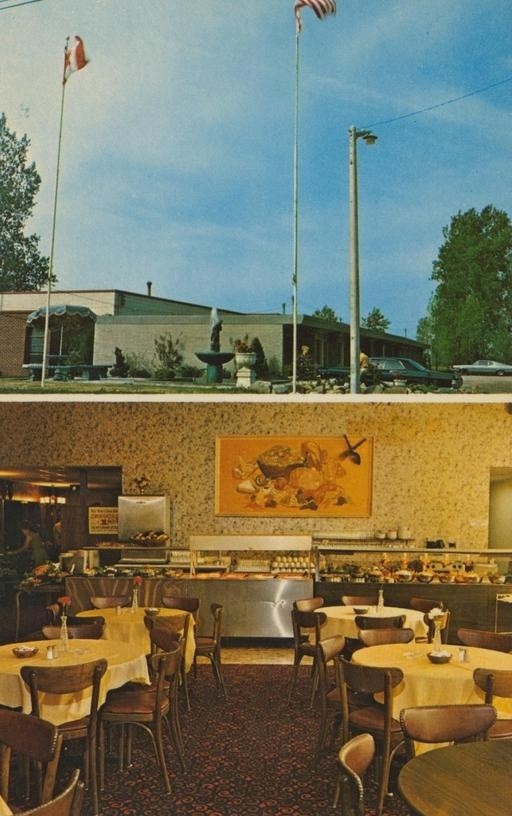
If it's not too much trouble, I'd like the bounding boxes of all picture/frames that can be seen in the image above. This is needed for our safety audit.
[210,428,376,522]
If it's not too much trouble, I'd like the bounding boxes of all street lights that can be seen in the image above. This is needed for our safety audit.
[348,124,377,394]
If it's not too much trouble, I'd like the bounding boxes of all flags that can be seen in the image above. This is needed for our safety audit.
[61,34,92,83]
[292,0,337,34]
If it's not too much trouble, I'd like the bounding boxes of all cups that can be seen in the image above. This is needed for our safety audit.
[169,549,224,565]
[236,556,316,575]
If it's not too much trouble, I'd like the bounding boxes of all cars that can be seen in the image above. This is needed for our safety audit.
[453,359,512,375]
[318,358,464,394]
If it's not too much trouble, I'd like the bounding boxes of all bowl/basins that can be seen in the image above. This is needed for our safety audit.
[12,647,40,659]
[144,608,161,617]
[425,540,456,549]
[320,565,508,584]
[426,653,452,663]
[352,606,369,614]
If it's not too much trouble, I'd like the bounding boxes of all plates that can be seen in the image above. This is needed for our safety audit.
[376,530,411,540]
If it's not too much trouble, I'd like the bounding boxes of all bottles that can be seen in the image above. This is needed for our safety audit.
[45,644,59,660]
[116,605,135,617]
[458,647,470,663]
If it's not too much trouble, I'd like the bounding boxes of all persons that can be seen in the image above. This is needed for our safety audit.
[0,581,71,646]
[360,349,369,374]
[5,519,50,568]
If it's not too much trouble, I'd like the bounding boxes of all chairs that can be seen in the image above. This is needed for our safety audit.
[1,568,511,816]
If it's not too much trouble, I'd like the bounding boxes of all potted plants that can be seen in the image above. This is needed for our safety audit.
[393,372,409,387]
[232,337,259,390]
